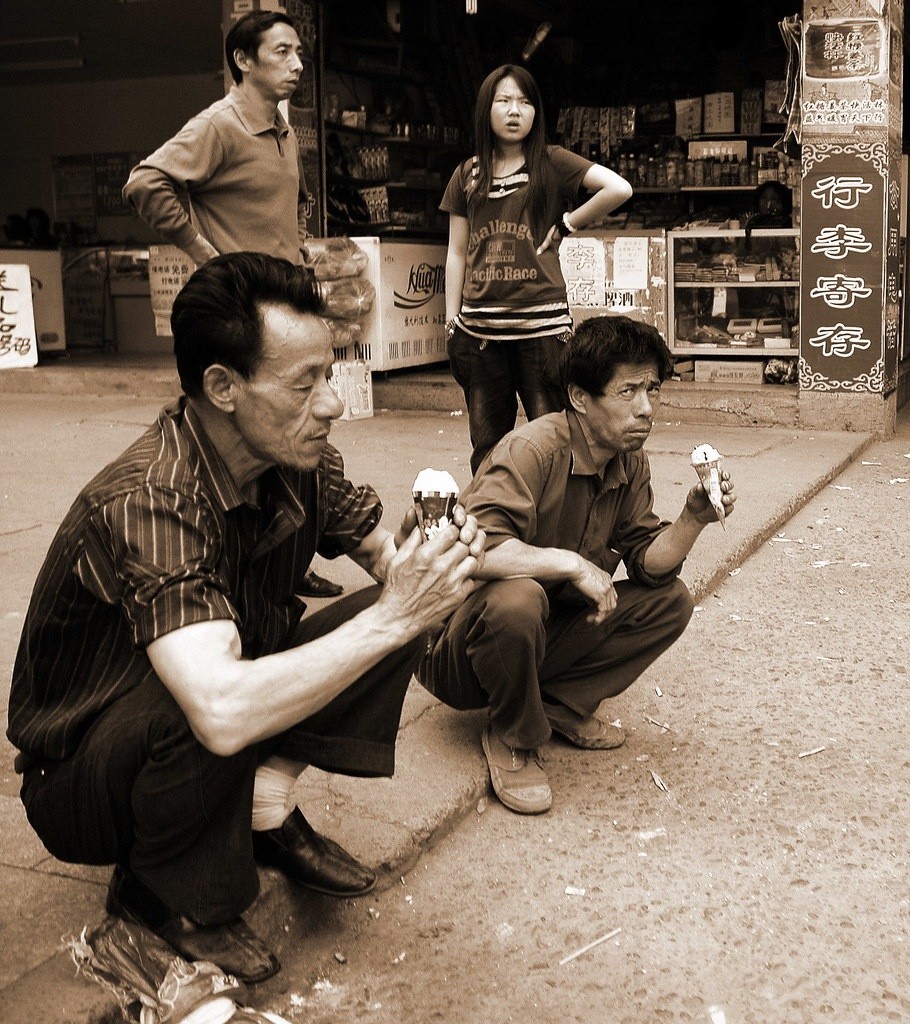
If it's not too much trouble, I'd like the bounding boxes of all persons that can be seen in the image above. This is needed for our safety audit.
[123,11,303,267]
[413,314,736,815]
[445,65,633,480]
[6,250,486,985]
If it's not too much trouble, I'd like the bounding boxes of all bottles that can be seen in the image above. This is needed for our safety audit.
[95,158,134,215]
[357,105,367,129]
[578,147,797,188]
[358,145,388,179]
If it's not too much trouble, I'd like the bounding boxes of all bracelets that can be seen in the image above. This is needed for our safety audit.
[550,212,576,243]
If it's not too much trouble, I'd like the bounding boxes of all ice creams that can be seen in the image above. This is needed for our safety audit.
[411,467,459,543]
[690,443,726,532]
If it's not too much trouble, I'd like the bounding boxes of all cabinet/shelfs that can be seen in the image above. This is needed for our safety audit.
[323,117,800,235]
[666,228,802,361]
[45,145,157,243]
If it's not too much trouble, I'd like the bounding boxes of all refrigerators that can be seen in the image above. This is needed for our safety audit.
[320,232,456,374]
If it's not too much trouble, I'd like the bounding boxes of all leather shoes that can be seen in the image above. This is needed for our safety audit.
[251,803,377,897]
[105,866,281,983]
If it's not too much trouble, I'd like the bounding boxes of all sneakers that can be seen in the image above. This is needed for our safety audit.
[481,722,552,814]
[540,701,626,748]
